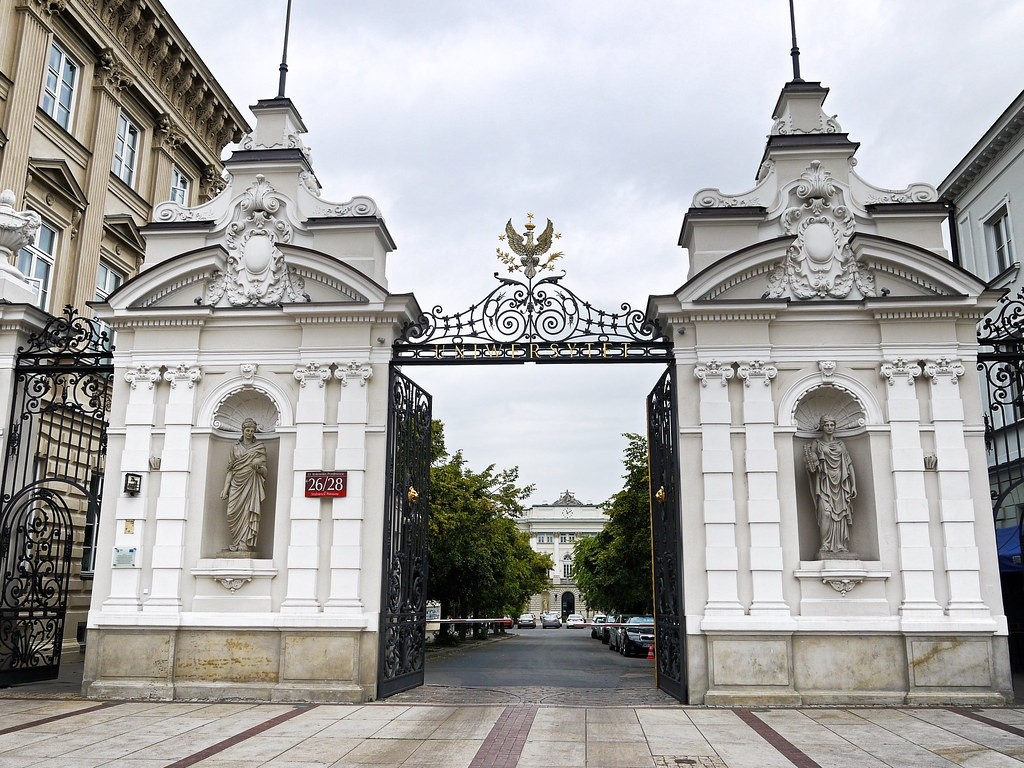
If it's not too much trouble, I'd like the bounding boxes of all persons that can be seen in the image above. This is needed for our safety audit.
[220,417,269,553]
[802,415,858,551]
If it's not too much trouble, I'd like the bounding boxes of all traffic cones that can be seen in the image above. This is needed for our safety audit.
[646,642,655,659]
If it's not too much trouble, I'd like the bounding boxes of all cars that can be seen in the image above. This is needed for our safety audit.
[542,611,563,628]
[591,615,655,657]
[566,614,586,629]
[517,613,537,629]
[495,615,515,629]
